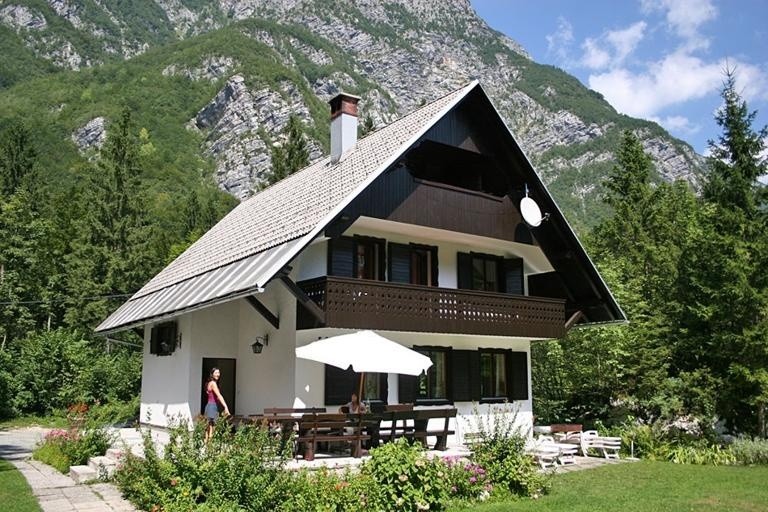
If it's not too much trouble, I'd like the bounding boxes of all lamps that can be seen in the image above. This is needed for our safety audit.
[250,333,269,354]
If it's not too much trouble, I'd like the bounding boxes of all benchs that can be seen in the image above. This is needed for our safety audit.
[227,403,458,462]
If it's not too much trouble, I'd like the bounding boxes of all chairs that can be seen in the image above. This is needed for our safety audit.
[524,429,623,472]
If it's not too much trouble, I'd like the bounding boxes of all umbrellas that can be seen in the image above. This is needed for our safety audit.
[295,330,435,457]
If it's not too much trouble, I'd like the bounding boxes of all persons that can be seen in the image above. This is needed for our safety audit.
[204,367,231,439]
[339,393,366,414]
[327,406,355,450]
